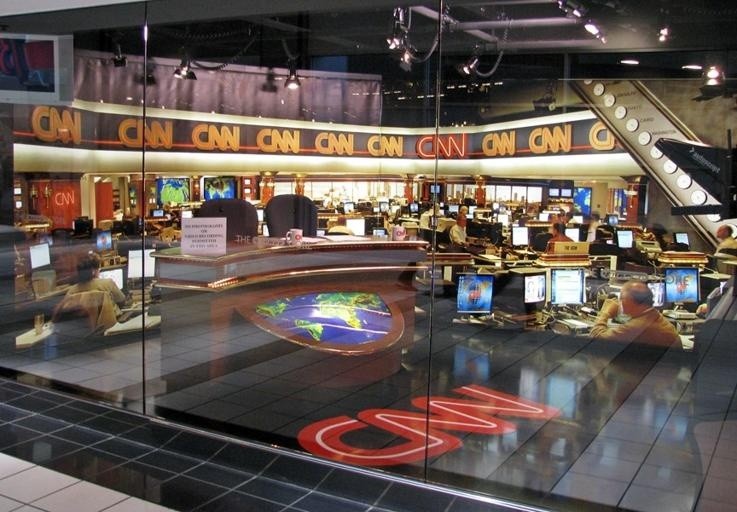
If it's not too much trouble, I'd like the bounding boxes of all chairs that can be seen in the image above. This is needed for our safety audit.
[52,291,119,354]
[534,233,554,254]
[198,200,259,243]
[264,195,317,237]
[589,243,626,271]
[417,227,450,250]
[365,216,385,238]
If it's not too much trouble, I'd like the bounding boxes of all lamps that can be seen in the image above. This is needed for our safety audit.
[384,6,410,52]
[458,55,479,75]
[397,45,415,69]
[283,74,301,90]
[173,62,197,80]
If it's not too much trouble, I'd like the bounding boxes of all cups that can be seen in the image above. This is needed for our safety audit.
[391,226,406,241]
[285,229,303,245]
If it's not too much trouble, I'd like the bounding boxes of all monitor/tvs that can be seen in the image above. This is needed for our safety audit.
[317,227,327,237]
[150,209,164,221]
[564,228,579,242]
[538,213,550,223]
[26,242,53,271]
[664,267,698,310]
[604,214,617,224]
[511,227,528,250]
[408,202,421,213]
[608,215,618,225]
[497,213,510,227]
[125,248,158,281]
[522,271,546,314]
[447,204,460,214]
[492,202,501,212]
[453,273,495,324]
[255,207,264,222]
[95,229,116,254]
[550,268,583,304]
[647,280,664,313]
[261,221,269,236]
[560,188,573,199]
[344,219,365,236]
[199,174,237,203]
[372,206,381,213]
[572,216,583,224]
[430,184,441,194]
[673,232,689,246]
[548,187,561,198]
[180,211,194,218]
[373,230,386,235]
[388,205,402,220]
[91,264,127,295]
[0,33,75,105]
[673,229,691,248]
[615,230,633,249]
[343,202,356,214]
[155,176,192,209]
[379,201,390,213]
[468,205,478,215]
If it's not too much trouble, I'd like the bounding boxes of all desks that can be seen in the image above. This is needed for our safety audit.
[423,246,518,284]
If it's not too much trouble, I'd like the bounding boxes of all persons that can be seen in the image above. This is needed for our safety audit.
[451,213,467,244]
[459,204,470,215]
[545,223,574,257]
[590,281,682,348]
[564,213,575,224]
[713,225,737,256]
[66,255,127,328]
[548,214,558,222]
